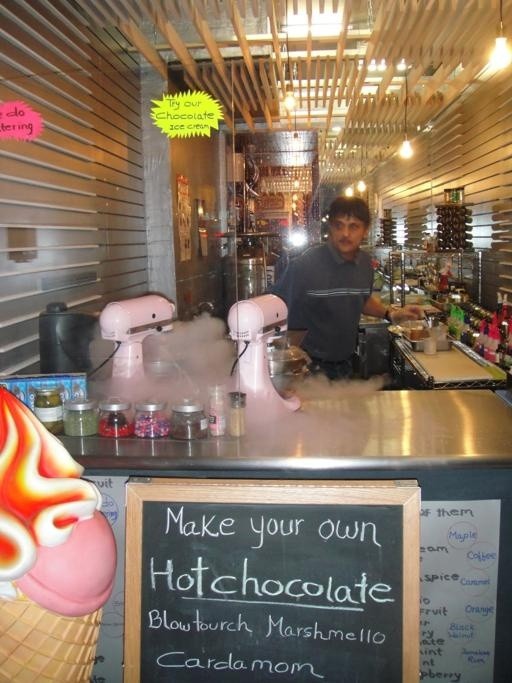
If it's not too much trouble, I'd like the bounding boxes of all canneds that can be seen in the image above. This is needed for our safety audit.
[34,389,64,435]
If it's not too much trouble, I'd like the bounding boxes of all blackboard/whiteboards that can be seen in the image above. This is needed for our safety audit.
[123,479,423,683]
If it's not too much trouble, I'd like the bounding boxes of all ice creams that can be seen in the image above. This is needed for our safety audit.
[0,386,117,683]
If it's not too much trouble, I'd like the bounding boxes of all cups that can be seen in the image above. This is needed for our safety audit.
[422,338,437,355]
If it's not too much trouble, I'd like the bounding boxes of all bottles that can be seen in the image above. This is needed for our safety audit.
[228,391,246,435]
[27,387,211,446]
[208,385,228,436]
[448,285,512,375]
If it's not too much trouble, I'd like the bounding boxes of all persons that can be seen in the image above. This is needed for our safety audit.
[267,196,427,380]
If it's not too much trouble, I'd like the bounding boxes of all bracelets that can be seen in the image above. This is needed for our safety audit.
[385,310,392,323]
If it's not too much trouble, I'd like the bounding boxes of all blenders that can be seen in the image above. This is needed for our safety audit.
[99,293,199,404]
[225,292,307,418]
